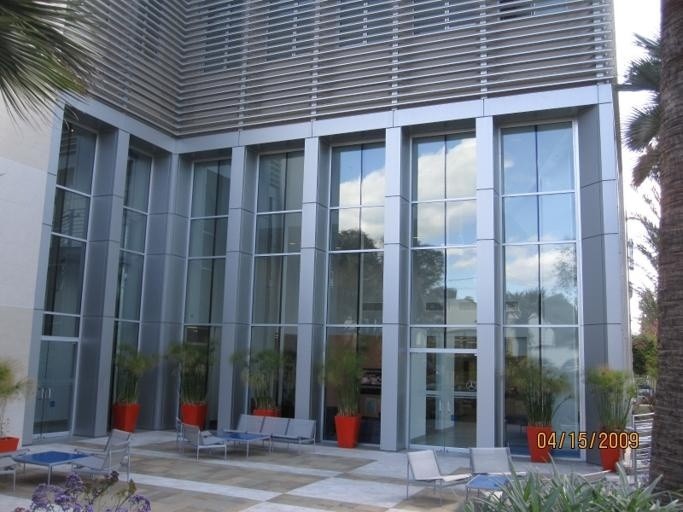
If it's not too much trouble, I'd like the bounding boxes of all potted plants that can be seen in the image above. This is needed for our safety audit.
[111,343,153,433]
[0,359,34,454]
[230,345,288,417]
[323,346,366,448]
[165,342,217,430]
[508,359,575,462]
[587,365,640,471]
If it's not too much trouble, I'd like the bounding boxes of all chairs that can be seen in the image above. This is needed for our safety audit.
[405,450,472,505]
[0,448,29,472]
[467,447,525,479]
[0,456,18,493]
[174,413,316,465]
[71,429,132,484]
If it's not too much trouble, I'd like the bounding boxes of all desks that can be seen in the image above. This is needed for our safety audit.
[465,474,509,504]
[14,450,97,487]
